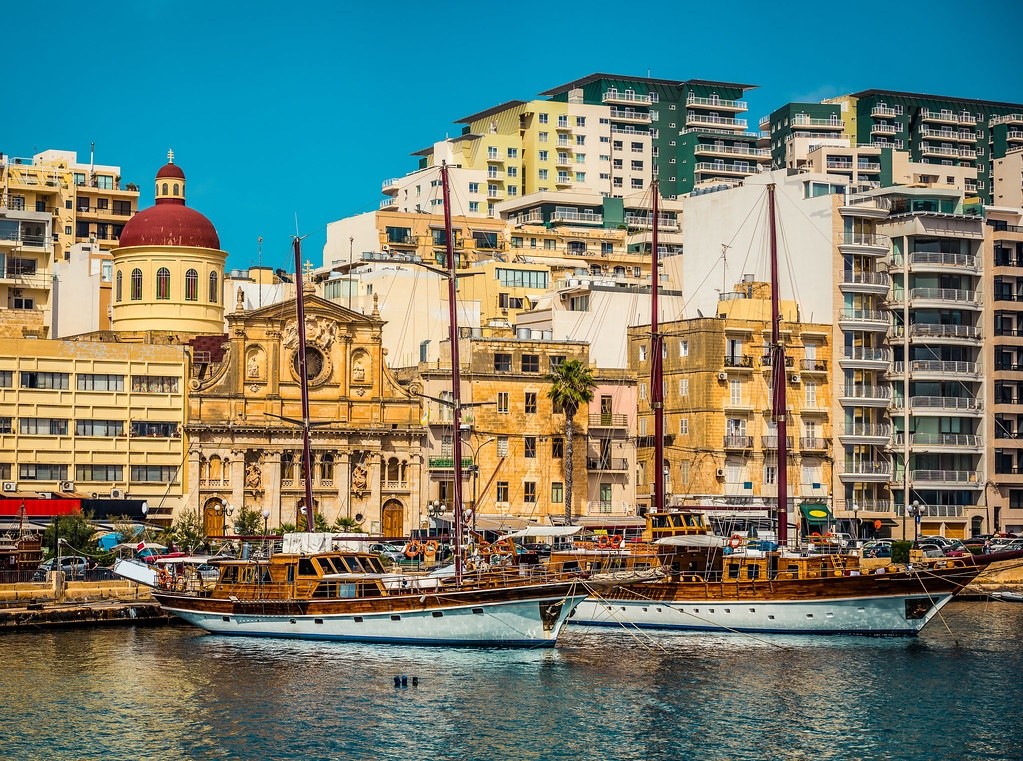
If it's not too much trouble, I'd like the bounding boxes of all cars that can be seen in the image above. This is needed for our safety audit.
[36,556,88,579]
[828,532,1023,558]
[368,542,576,563]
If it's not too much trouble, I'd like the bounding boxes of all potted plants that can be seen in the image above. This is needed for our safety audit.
[461,252,468,269]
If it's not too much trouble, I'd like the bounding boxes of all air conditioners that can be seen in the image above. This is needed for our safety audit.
[602,264,609,270]
[589,459,601,470]
[15,288,22,296]
[626,265,633,271]
[791,374,800,384]
[382,244,390,250]
[3,482,17,492]
[62,480,74,491]
[110,488,124,500]
[716,468,725,477]
[88,492,99,499]
[719,372,727,381]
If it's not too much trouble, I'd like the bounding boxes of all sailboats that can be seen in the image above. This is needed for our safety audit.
[146,234,599,650]
[491,180,1023,635]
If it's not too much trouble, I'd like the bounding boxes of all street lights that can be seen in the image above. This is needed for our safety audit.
[452,503,473,540]
[262,510,270,549]
[428,500,445,537]
[907,500,926,550]
[852,505,860,539]
[460,437,496,535]
[214,499,235,536]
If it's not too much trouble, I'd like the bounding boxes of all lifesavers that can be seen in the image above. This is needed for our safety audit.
[610,534,622,549]
[810,532,822,545]
[598,535,609,548]
[424,540,439,556]
[730,535,742,548]
[405,540,421,557]
[158,570,168,584]
[823,532,834,546]
[495,541,509,556]
[479,541,491,553]
